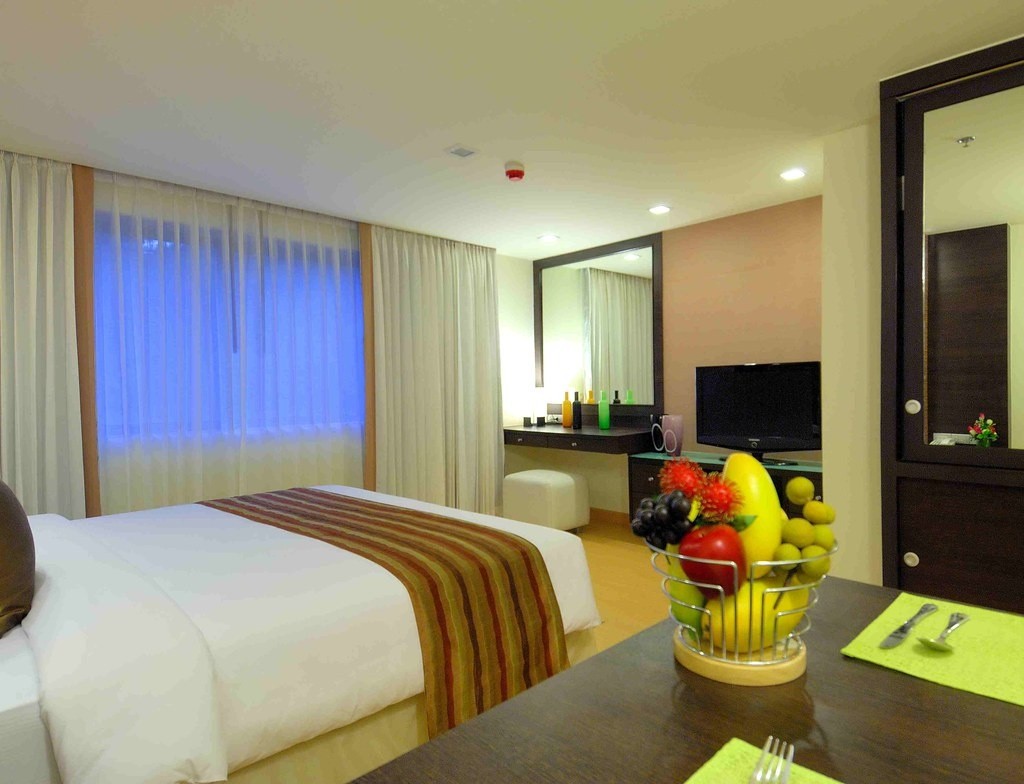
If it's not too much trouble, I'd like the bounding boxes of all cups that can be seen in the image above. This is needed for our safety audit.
[662,414,683,456]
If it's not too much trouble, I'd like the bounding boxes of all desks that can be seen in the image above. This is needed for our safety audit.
[502,425,653,524]
[352,575,1024,784]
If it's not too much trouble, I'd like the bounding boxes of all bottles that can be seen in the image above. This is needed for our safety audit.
[572,392,582,429]
[625,390,635,404]
[598,391,610,430]
[613,390,620,404]
[562,393,571,427]
[587,390,595,404]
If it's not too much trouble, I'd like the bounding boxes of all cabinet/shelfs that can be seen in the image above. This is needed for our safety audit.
[631,451,823,541]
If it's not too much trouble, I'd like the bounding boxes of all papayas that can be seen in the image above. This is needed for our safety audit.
[719,451,783,581]
[699,573,810,652]
[665,542,709,643]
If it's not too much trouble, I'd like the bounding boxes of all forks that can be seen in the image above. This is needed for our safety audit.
[748,735,794,784]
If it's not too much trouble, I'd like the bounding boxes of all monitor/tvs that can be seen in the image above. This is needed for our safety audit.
[697,361,822,465]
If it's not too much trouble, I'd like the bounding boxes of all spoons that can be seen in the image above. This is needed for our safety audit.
[916,612,970,651]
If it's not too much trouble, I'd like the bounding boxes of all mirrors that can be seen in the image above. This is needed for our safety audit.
[534,232,664,416]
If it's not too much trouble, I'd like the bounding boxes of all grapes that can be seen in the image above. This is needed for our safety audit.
[631,490,698,549]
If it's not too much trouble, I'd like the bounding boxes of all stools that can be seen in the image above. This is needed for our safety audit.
[502,470,591,534]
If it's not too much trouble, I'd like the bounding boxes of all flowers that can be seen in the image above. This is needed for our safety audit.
[968,412,998,440]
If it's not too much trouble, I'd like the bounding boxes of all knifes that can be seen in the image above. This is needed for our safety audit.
[878,604,937,649]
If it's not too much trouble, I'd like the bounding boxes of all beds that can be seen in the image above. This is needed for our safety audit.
[0,485,600,784]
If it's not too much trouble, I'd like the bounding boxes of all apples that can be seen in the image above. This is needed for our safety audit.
[679,524,747,599]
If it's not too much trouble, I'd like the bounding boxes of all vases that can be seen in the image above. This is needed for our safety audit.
[976,437,990,447]
